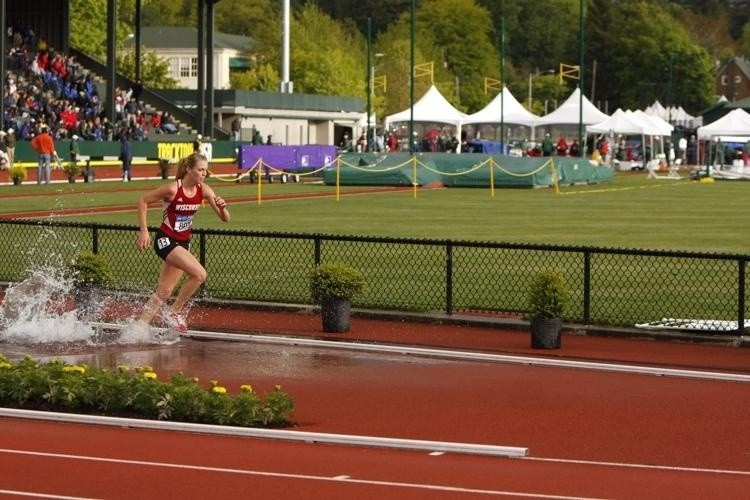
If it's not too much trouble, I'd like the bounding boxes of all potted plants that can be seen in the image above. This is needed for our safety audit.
[67,253,116,314]
[307,262,365,335]
[521,273,567,349]
[63,164,81,183]
[11,163,27,185]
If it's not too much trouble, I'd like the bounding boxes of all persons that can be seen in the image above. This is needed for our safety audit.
[194,133,203,154]
[0,132,10,171]
[5,128,16,162]
[70,135,79,166]
[253,132,262,145]
[232,119,240,141]
[135,154,229,335]
[120,135,132,182]
[0,23,180,142]
[31,129,57,184]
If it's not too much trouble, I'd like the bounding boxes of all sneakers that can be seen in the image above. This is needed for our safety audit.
[164,311,187,333]
